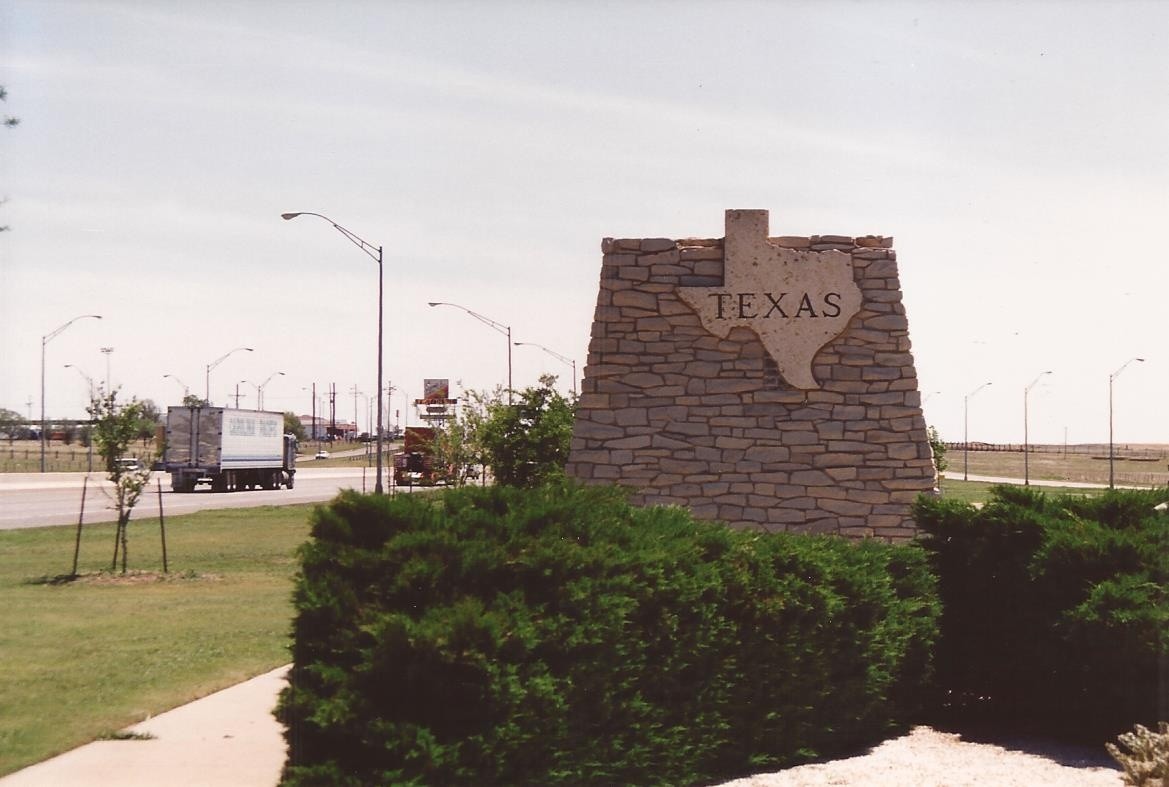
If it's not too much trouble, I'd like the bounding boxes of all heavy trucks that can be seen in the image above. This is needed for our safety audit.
[164,406,296,493]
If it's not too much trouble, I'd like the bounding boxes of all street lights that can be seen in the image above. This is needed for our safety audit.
[301,388,318,442]
[164,373,188,404]
[64,365,92,472]
[101,347,115,415]
[282,212,385,495]
[242,380,260,410]
[42,314,102,472]
[1023,371,1054,485]
[1110,357,1143,488]
[515,342,576,407]
[257,372,285,410]
[963,382,993,478]
[205,348,254,406]
[428,301,511,407]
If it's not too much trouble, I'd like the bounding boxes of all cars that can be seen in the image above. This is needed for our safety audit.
[316,451,328,459]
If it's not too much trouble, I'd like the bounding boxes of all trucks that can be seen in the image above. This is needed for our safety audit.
[394,426,455,485]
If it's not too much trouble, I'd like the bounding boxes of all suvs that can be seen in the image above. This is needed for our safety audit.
[462,455,483,479]
[112,458,140,479]
